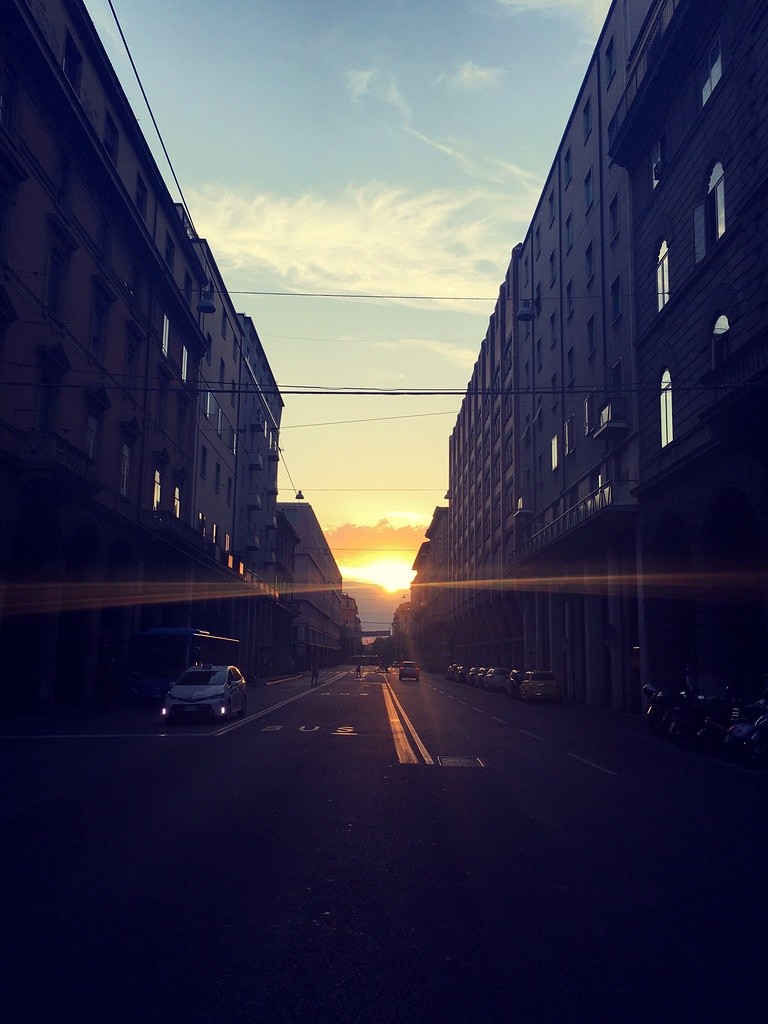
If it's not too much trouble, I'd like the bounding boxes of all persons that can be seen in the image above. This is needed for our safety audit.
[311,663,319,687]
[356,664,361,677]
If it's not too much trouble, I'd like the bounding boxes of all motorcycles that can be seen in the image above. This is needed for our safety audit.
[641,666,767,768]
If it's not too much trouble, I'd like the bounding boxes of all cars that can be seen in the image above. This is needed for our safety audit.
[392,661,400,669]
[506,669,528,700]
[162,663,248,725]
[519,669,563,704]
[398,660,421,681]
[445,662,488,689]
[484,668,513,694]
